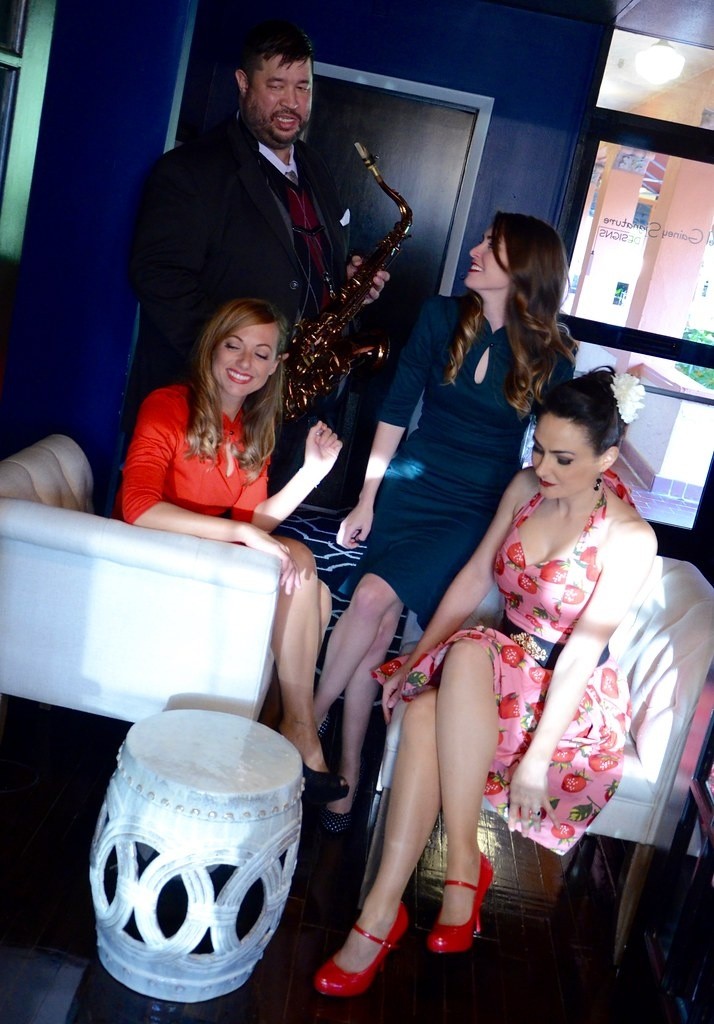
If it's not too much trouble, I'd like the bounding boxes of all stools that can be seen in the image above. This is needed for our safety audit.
[89,709,306,1003]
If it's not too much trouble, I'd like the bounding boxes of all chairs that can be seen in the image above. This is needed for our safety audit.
[357,555,714,966]
[0,435,275,795]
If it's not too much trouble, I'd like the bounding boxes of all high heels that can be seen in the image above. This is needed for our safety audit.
[303,762,350,804]
[426,848,496,957]
[312,893,410,998]
[320,775,363,835]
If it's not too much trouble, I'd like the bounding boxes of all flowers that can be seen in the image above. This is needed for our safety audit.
[610,373,646,424]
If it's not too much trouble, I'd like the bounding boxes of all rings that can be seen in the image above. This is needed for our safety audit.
[531,811,541,816]
[316,429,323,436]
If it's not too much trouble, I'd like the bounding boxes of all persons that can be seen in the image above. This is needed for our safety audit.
[301,361,657,998]
[311,211,579,836]
[124,292,349,802]
[111,26,393,486]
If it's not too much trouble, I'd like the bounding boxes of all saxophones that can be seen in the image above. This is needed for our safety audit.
[281,139,416,419]
